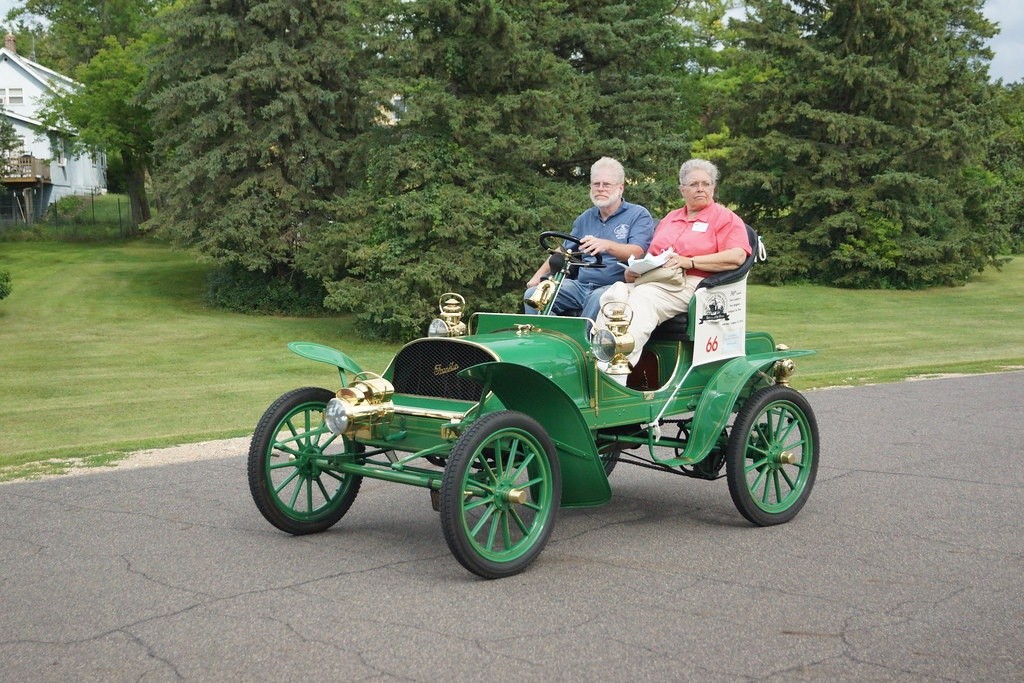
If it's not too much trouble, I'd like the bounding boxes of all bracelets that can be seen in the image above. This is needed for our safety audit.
[691,258,694,268]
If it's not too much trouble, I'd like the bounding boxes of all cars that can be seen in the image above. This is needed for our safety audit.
[246,224,820,580]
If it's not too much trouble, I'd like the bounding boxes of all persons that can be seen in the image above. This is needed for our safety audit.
[524,156,655,340]
[590,159,752,387]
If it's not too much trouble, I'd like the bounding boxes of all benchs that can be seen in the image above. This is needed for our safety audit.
[569,223,759,341]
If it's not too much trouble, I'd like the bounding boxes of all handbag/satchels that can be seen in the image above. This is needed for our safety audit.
[635,265,687,291]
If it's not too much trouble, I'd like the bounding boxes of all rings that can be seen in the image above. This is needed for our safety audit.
[675,259,677,264]
